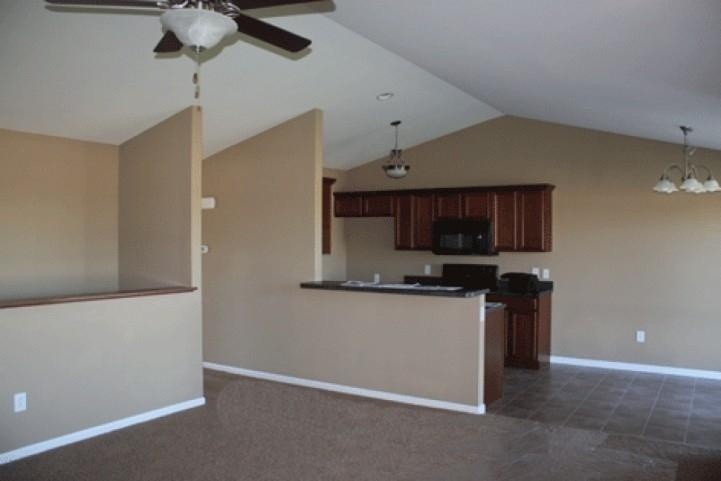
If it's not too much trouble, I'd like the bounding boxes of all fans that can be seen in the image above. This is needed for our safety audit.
[46,0,335,57]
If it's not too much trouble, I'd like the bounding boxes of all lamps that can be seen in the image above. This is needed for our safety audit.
[381,120,412,180]
[160,2,240,84]
[652,125,721,195]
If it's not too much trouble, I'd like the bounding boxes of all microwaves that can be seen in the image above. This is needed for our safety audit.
[430,220,493,257]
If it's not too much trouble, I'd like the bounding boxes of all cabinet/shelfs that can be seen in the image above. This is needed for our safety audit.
[436,191,493,221]
[504,290,553,368]
[493,190,553,252]
[397,194,434,248]
[334,195,394,219]
